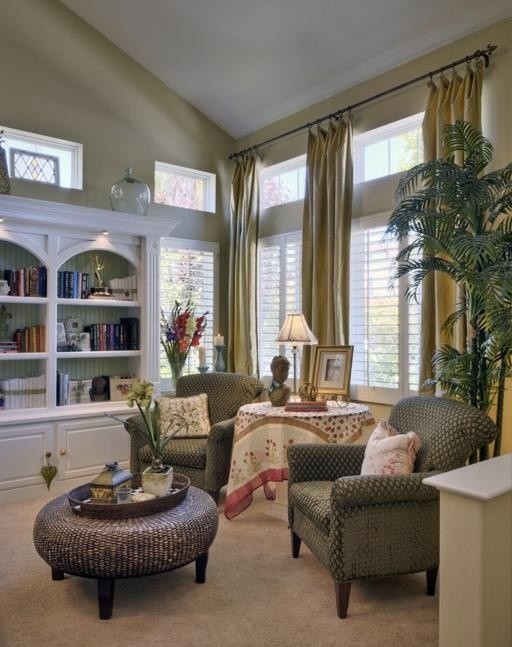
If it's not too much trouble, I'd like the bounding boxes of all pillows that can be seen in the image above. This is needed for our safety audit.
[360,419,422,476]
[155,393,211,439]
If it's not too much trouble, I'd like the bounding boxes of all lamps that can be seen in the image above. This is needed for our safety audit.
[273,313,318,395]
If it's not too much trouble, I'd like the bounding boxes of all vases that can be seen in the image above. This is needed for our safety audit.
[109,167,150,216]
[169,358,184,389]
[141,465,175,496]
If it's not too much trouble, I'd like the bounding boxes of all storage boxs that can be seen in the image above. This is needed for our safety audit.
[91,470,133,503]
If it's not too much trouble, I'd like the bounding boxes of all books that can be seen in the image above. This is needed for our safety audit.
[106,273,138,301]
[0,372,47,410]
[57,370,71,406]
[2,323,46,353]
[84,317,139,351]
[57,270,91,300]
[3,266,48,298]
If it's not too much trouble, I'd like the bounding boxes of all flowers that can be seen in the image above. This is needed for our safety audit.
[160,295,209,382]
[102,379,187,472]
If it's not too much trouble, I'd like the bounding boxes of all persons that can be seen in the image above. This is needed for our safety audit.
[268,355,292,407]
[89,378,110,401]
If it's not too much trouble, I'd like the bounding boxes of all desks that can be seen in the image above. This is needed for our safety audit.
[223,396,376,521]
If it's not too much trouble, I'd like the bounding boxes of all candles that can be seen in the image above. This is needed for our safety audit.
[214,333,224,344]
[199,346,207,367]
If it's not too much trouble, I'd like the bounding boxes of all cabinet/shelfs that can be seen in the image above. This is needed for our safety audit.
[0,193,180,504]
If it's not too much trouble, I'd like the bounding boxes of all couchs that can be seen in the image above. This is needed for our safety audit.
[124,372,267,507]
[288,398,499,619]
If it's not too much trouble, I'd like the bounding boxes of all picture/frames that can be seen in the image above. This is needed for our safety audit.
[312,344,354,396]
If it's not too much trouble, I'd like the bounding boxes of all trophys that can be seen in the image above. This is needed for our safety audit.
[91,255,110,295]
